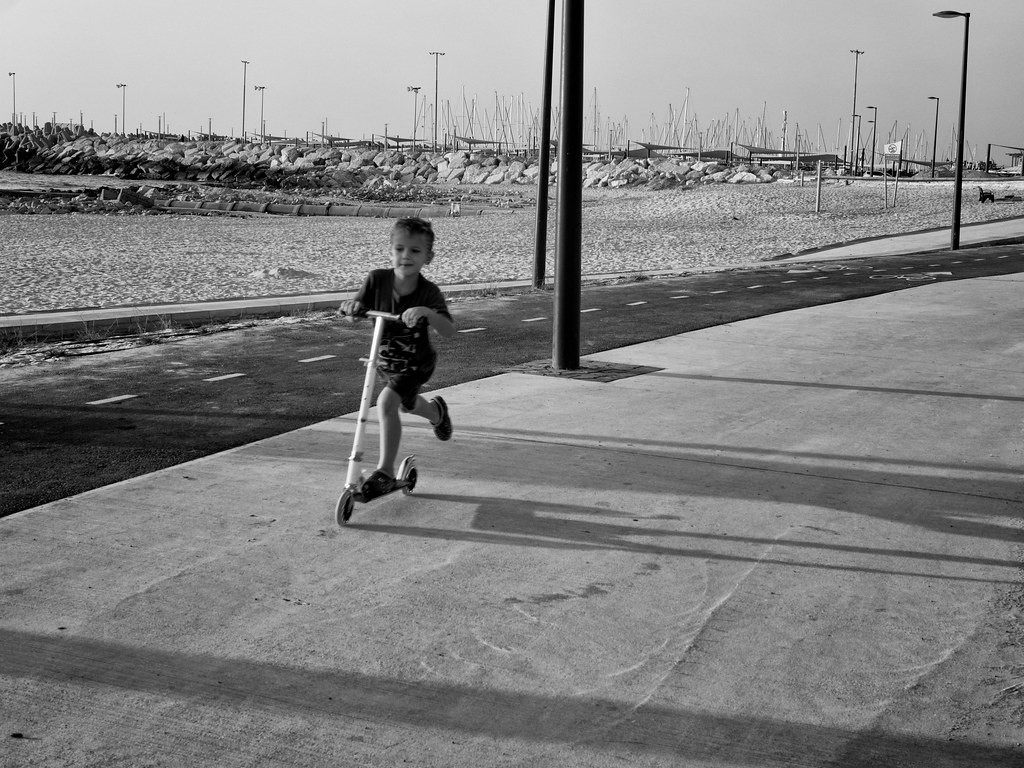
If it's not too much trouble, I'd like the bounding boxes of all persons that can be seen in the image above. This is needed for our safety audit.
[342,219,455,487]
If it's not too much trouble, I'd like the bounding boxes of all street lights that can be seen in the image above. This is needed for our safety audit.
[851,97,942,178]
[931,6,974,252]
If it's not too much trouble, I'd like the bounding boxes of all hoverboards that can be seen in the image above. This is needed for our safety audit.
[333,308,416,528]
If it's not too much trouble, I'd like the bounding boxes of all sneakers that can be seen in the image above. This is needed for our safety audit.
[431,396,452,441]
[362,471,397,493]
